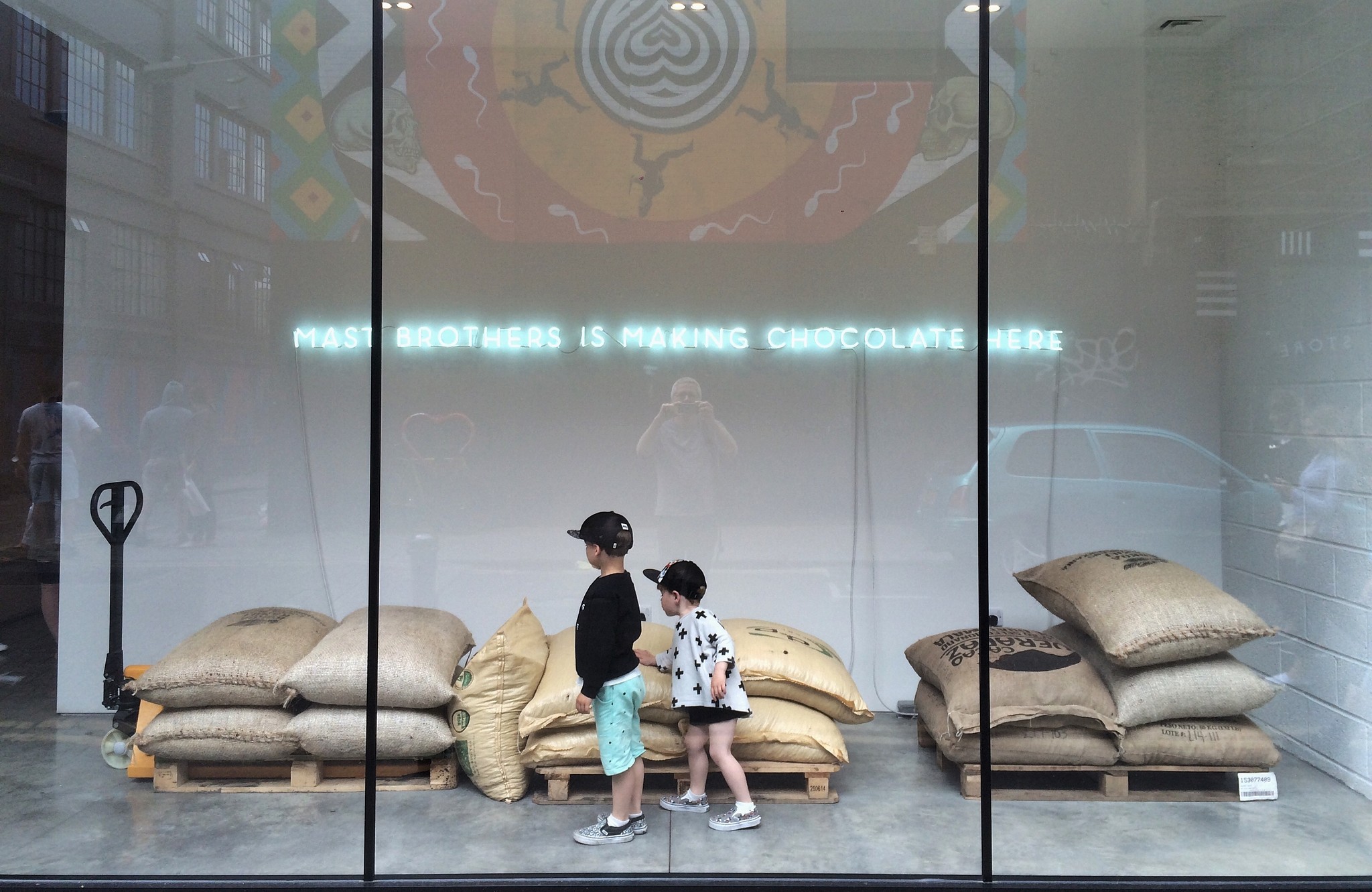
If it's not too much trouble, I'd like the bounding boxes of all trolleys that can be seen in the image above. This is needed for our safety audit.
[403,413,476,608]
[91,481,164,778]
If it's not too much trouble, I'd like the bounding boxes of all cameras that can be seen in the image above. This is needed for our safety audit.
[678,403,697,414]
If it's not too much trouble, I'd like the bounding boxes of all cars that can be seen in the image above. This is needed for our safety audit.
[949,424,1286,578]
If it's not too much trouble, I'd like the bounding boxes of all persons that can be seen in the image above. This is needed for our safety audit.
[637,377,738,517]
[1255,404,1342,685]
[567,511,649,845]
[633,559,761,831]
[14,375,211,644]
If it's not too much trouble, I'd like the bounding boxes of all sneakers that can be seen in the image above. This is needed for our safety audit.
[709,804,761,831]
[572,817,635,844]
[660,789,710,813]
[598,812,648,835]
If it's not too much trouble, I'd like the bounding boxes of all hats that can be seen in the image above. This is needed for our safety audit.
[642,559,707,600]
[567,511,633,549]
